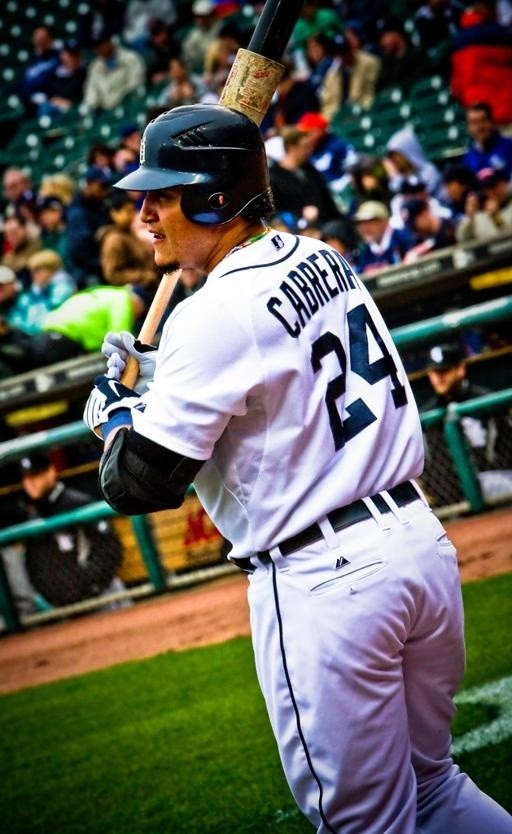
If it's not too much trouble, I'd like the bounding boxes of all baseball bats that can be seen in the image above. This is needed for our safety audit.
[94,0,306,441]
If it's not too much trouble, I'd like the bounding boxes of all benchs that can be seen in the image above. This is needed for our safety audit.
[116,492,225,585]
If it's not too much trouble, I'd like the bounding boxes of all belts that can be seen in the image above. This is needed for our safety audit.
[230,478,420,572]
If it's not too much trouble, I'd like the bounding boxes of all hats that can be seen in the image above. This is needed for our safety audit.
[420,343,470,371]
[12,448,51,481]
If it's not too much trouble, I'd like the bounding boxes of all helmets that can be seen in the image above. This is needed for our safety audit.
[113,102,271,226]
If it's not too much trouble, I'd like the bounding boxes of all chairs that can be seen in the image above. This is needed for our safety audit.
[1,0,512,382]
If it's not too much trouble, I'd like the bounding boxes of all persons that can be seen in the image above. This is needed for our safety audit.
[83,104,510,831]
[2,1,512,616]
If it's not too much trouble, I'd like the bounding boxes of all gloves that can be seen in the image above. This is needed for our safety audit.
[82,331,158,439]
